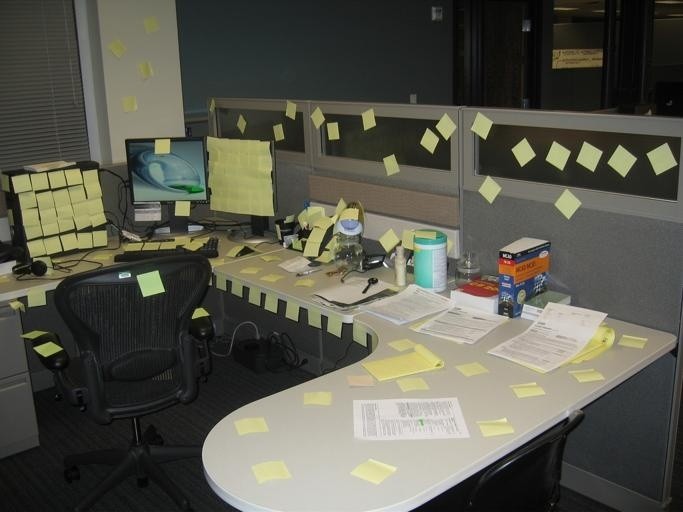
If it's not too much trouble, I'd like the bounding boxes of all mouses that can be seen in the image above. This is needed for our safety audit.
[236,246,254,257]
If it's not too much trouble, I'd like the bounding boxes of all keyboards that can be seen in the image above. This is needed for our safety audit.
[114,238,219,262]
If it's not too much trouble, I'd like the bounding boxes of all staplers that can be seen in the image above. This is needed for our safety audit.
[362,254,385,269]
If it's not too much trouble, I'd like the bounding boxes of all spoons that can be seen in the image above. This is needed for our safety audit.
[362,278,378,294]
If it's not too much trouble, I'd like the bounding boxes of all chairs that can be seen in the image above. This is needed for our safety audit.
[29,254,218,512]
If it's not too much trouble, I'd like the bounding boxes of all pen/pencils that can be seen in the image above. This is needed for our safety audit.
[298,267,323,276]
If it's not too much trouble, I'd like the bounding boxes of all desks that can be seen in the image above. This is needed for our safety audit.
[1,222,292,464]
[199,245,680,512]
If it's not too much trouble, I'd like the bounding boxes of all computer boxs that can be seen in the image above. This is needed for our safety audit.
[2,161,107,262]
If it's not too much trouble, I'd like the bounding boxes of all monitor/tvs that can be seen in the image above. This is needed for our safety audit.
[125,138,211,234]
[204,137,278,245]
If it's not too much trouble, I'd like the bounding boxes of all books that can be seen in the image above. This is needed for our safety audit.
[497,236,551,320]
[360,343,444,381]
[521,290,572,315]
[449,272,501,315]
[312,287,399,311]
[520,311,544,322]
[563,326,615,365]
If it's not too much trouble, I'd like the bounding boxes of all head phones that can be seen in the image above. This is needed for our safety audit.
[12,261,47,276]
